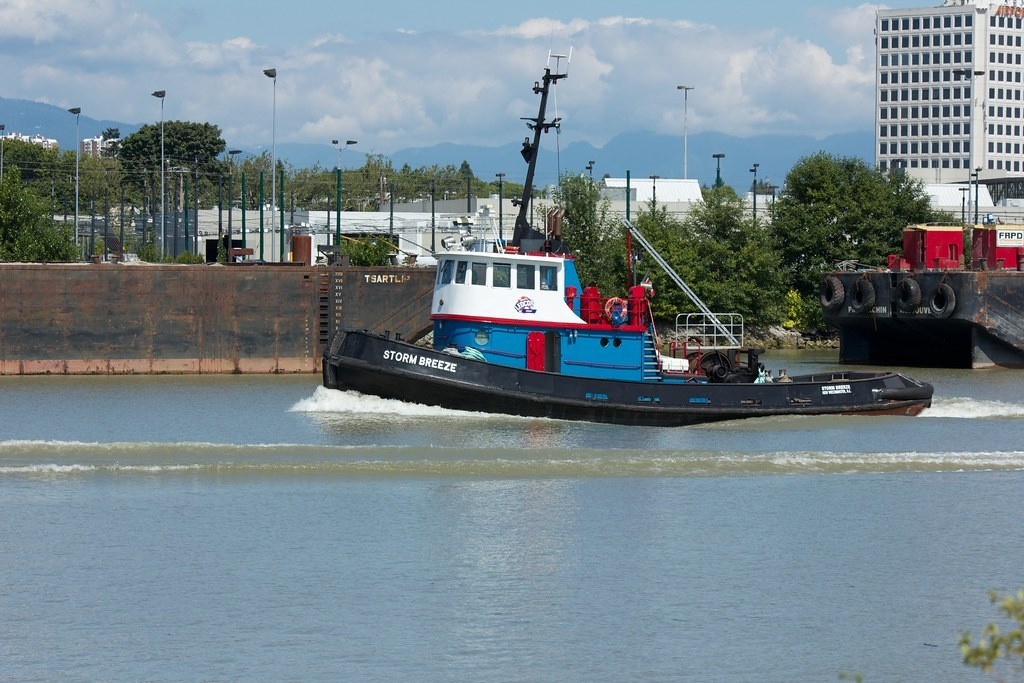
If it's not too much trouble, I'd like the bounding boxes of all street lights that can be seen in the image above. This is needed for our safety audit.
[952,70,986,228]
[959,187,969,226]
[749,163,759,220]
[263,68,277,262]
[649,175,660,218]
[330,139,357,245]
[151,89,166,260]
[0,123,5,184]
[713,153,726,188]
[495,172,506,239]
[67,107,81,247]
[228,149,242,263]
[585,160,594,230]
[971,166,982,225]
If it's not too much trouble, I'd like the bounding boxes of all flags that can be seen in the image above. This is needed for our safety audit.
[645,275,655,297]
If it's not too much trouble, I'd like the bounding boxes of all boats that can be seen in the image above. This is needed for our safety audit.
[819,222,1024,370]
[323,52,933,430]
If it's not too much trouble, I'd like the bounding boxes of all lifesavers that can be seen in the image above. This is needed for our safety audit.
[927,283,957,321]
[604,294,628,326]
[819,274,846,317]
[892,275,921,312]
[505,244,520,254]
[848,279,877,314]
